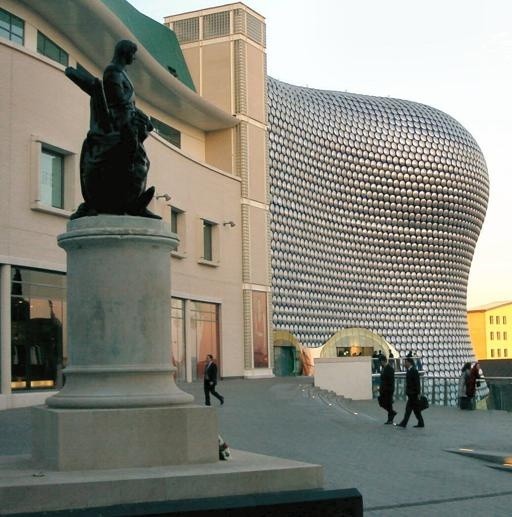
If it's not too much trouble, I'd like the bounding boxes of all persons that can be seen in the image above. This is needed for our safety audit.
[414,353,422,371]
[388,350,394,367]
[378,349,385,373]
[394,359,425,427]
[468,362,481,409]
[102,38,162,219]
[203,354,225,406]
[373,350,380,373]
[377,354,397,424]
[405,351,412,357]
[458,362,472,409]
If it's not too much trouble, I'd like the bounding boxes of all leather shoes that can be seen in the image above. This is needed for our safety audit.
[413,424,424,427]
[393,410,397,420]
[384,421,393,424]
[396,424,406,427]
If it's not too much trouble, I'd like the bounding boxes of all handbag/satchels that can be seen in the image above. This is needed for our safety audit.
[378,395,395,407]
[419,395,429,411]
